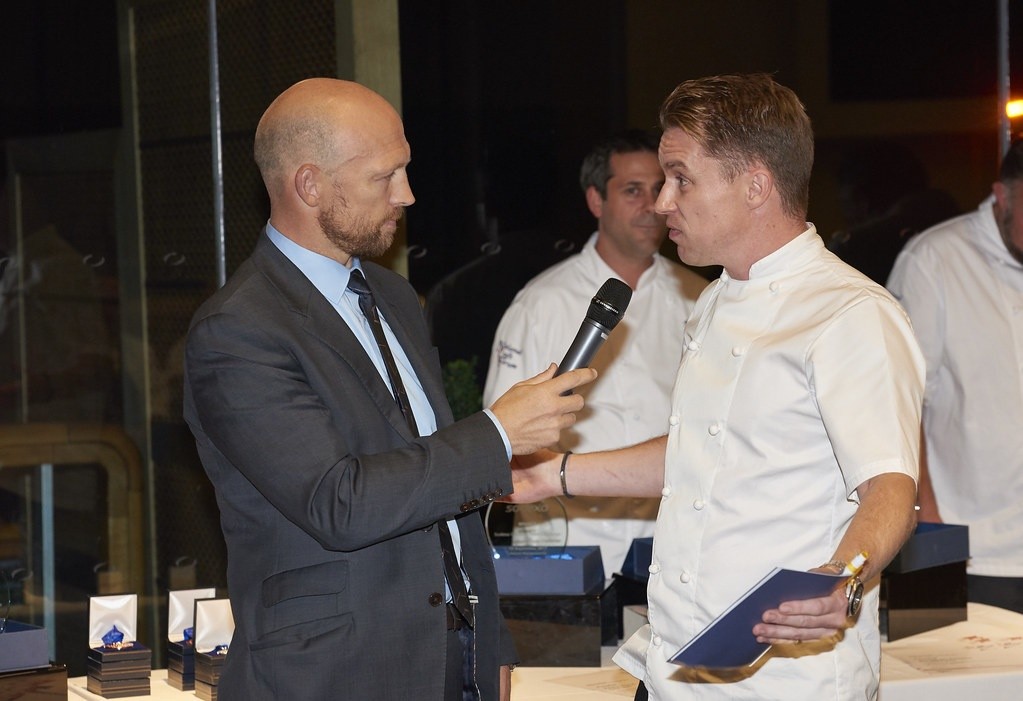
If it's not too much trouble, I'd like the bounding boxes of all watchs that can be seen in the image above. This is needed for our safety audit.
[819,560,864,618]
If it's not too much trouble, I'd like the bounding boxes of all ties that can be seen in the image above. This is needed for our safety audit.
[346,268,474,627]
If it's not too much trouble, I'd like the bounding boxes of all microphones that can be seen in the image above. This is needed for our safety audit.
[552,277,632,397]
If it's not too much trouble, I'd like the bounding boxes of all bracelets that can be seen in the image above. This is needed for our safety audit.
[507,664,516,671]
[560,451,575,499]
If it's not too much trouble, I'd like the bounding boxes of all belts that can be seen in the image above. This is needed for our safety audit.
[445,603,468,631]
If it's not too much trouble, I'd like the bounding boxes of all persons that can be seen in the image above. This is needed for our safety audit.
[483,74,926,701]
[886,139,1023,616]
[182,77,599,701]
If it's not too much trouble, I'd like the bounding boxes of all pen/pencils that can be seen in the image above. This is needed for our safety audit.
[842,550,870,577]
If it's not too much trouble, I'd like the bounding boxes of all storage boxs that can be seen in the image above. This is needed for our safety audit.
[885,522,972,578]
[487,542,605,596]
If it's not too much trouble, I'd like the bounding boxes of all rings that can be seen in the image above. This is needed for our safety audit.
[217,647,229,654]
[795,639,802,645]
[105,641,135,651]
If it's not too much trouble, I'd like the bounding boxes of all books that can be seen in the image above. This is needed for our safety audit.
[666,567,853,668]
